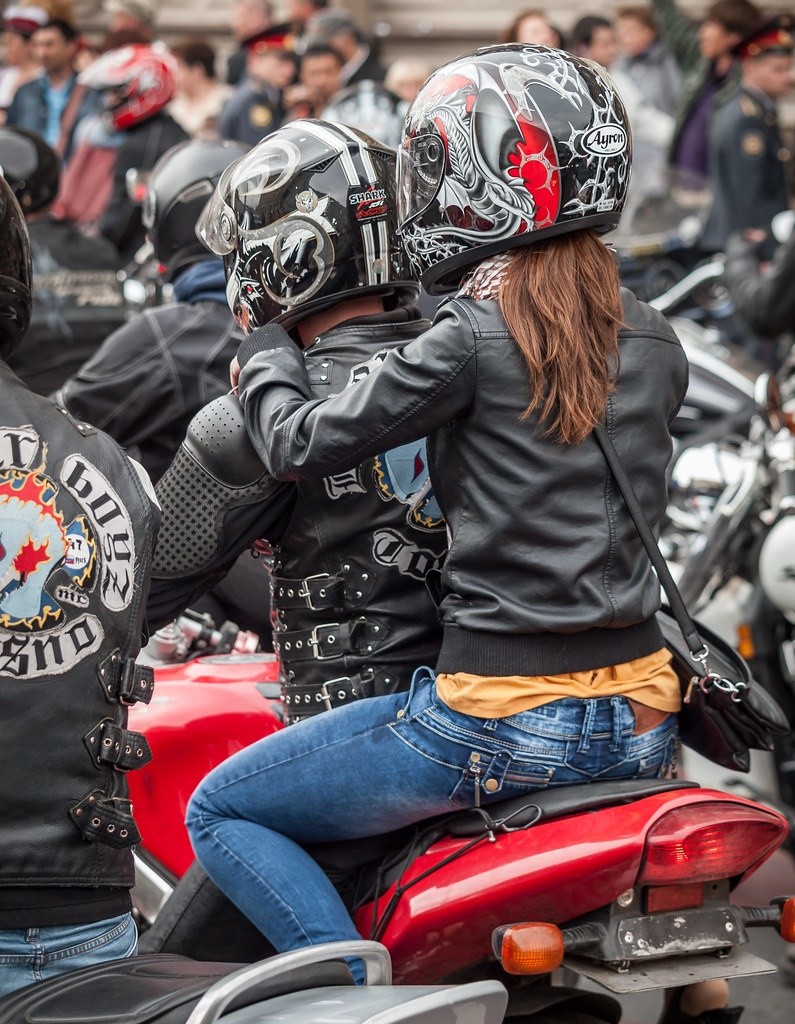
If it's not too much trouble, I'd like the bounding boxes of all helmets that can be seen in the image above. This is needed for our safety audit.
[396,43,634,296]
[76,46,175,134]
[195,118,421,335]
[0,175,32,362]
[0,123,61,215]
[142,137,254,282]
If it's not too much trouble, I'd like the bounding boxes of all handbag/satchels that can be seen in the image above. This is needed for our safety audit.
[655,603,790,774]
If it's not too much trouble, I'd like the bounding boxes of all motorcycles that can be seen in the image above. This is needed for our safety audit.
[127,606,795,1024]
[0,939,509,1024]
[1,126,172,397]
[605,160,795,855]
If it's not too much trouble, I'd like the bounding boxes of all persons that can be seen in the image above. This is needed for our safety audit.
[0,0,795,985]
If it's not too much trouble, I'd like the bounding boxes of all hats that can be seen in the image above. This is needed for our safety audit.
[727,14,795,59]
[240,20,305,60]
